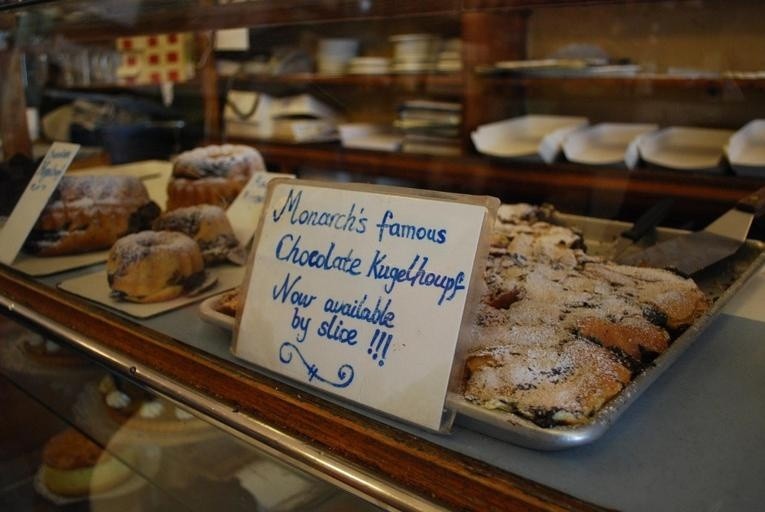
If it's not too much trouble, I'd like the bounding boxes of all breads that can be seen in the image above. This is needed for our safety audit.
[167,145,265,208]
[39,428,139,495]
[106,230,206,303]
[29,177,152,256]
[464,203,707,426]
[152,205,244,262]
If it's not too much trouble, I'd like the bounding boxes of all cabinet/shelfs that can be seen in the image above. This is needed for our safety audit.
[1,160,765,511]
[62,0,764,240]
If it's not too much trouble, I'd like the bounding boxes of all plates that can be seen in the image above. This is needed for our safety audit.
[197,205,763,451]
